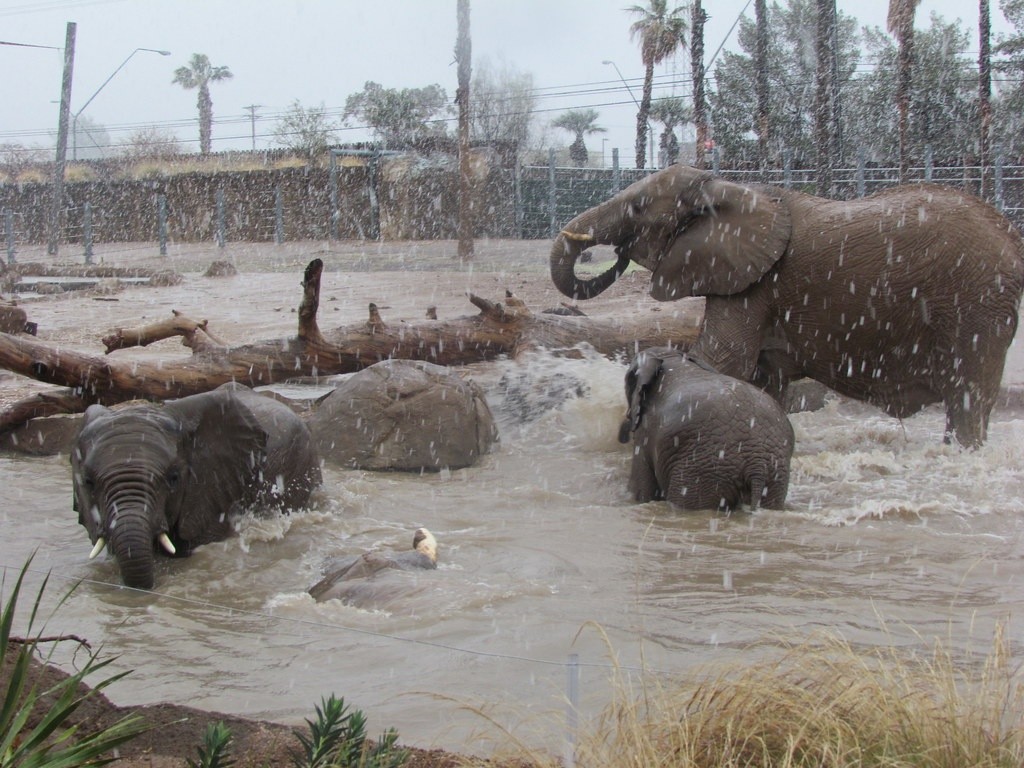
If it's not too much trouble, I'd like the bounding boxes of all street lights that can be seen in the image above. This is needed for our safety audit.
[601,59,655,170]
[73,47,171,162]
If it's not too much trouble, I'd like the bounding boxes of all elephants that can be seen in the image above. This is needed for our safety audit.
[548,164,1023,511]
[69,387,324,593]
